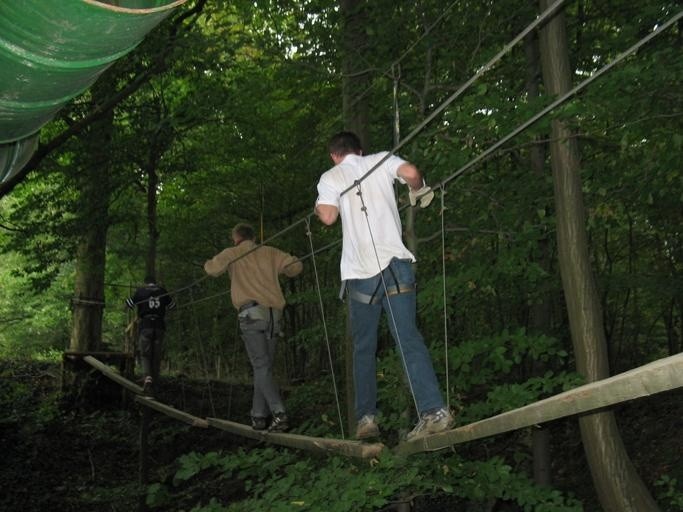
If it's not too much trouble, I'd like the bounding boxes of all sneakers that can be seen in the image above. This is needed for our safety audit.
[268,412,288,433]
[357,422,380,440]
[405,407,455,439]
[250,417,265,430]
[143,376,153,392]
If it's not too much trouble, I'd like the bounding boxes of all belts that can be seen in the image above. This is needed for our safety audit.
[239,300,257,312]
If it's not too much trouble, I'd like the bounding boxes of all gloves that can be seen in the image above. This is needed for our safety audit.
[408,178,435,208]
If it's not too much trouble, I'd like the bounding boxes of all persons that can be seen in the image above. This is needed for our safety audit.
[203,220,304,433]
[314,129,456,442]
[124,275,177,393]
